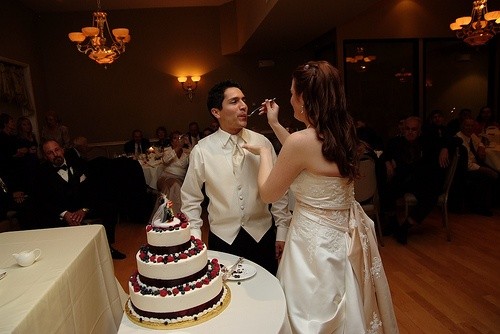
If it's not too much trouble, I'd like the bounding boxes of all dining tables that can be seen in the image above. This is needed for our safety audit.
[114,249,292,334]
[117,154,169,189]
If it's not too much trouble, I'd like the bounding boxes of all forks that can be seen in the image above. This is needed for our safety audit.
[241,98,275,117]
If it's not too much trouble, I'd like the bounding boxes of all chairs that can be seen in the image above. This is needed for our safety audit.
[405,148,459,239]
[352,158,383,248]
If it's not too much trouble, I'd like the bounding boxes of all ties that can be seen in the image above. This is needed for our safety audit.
[229,135,246,176]
[137,143,142,154]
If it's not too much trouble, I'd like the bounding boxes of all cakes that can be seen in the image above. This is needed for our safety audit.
[128,193,227,324]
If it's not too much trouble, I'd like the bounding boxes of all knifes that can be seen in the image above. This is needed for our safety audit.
[224,257,244,280]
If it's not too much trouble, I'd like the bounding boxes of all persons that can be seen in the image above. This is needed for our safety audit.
[180,81,293,277]
[351,107,499,246]
[0,112,220,259]
[241,61,399,334]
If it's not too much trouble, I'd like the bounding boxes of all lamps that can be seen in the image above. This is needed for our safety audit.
[450,0,500,46]
[67,0,131,72]
[177,76,201,103]
[345,47,376,72]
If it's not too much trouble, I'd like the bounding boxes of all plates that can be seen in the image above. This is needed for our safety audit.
[224,264,256,280]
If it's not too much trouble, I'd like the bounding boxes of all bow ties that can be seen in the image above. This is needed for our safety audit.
[52,164,67,172]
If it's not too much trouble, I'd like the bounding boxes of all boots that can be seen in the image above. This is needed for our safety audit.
[381,216,414,246]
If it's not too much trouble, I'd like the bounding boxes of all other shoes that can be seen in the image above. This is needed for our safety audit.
[109,245,127,260]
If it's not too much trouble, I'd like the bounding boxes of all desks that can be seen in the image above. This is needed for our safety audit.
[0,224,128,334]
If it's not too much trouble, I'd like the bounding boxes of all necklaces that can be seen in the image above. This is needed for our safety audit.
[306,124,311,129]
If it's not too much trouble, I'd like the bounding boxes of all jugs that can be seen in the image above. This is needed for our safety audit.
[11,248,42,267]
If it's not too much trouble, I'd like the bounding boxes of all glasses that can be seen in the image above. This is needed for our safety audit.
[405,126,417,131]
